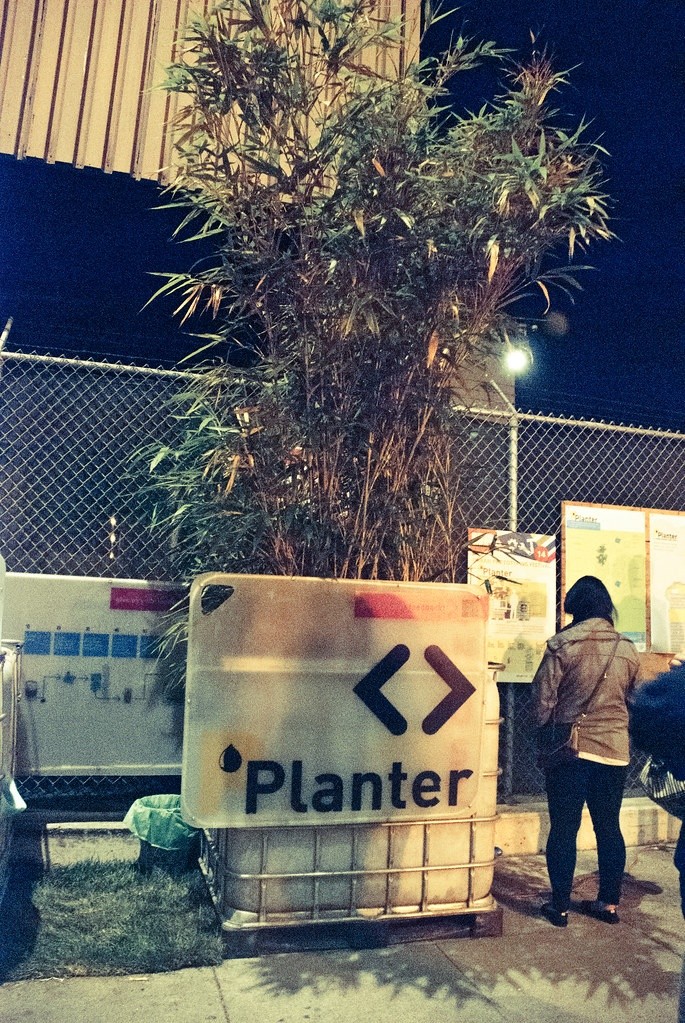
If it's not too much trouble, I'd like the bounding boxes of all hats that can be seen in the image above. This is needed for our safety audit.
[563,576,613,614]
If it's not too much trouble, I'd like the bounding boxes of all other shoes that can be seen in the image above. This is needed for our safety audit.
[579,899,621,924]
[541,903,568,927]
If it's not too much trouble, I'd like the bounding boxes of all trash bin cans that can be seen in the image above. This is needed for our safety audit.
[136,793,202,879]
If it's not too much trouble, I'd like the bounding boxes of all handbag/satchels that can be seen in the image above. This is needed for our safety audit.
[638,753,685,821]
[535,722,579,767]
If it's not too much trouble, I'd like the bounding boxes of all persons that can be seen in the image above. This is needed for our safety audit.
[528,575,643,927]
[627,651,685,1023]
